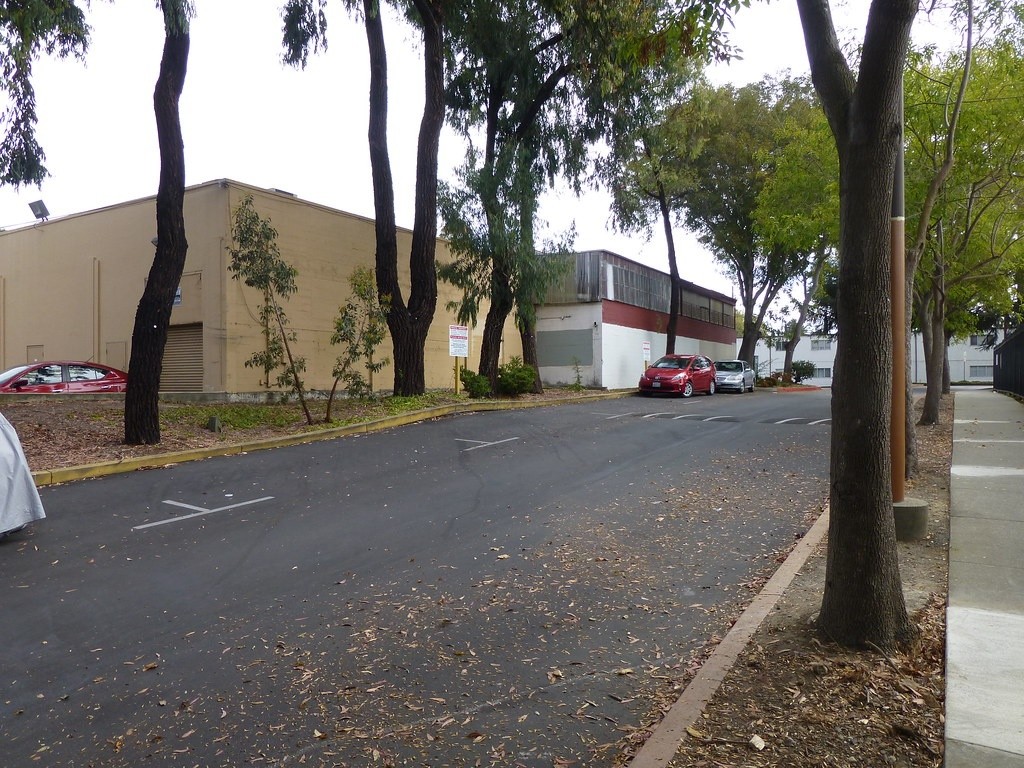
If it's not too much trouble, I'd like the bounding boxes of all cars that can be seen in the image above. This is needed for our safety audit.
[713,360,756,393]
[639,354,716,396]
[0,361,128,394]
